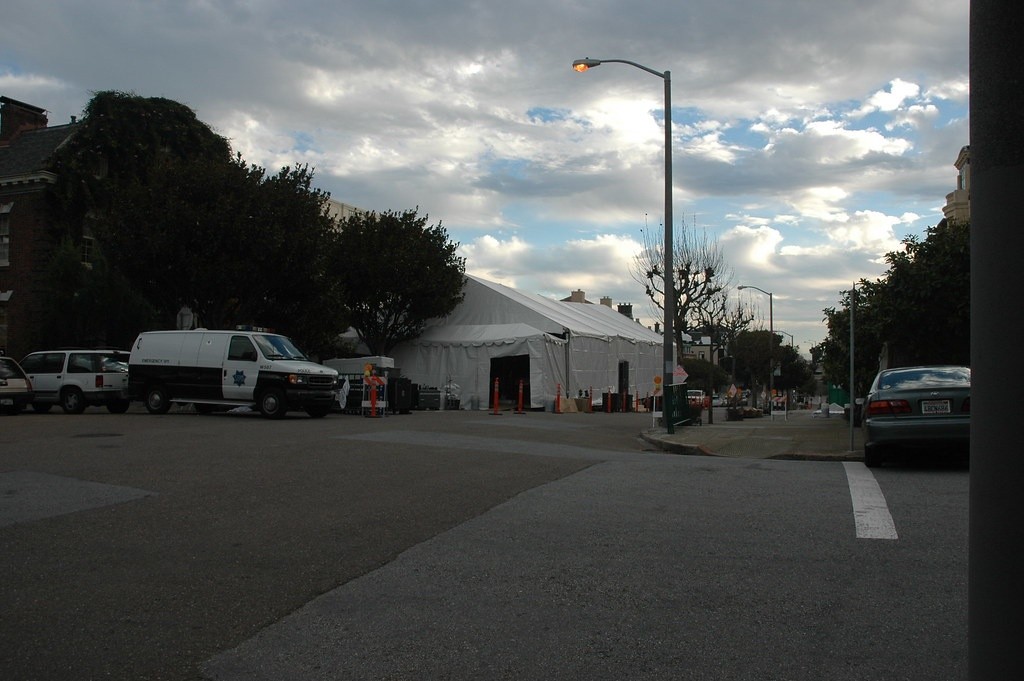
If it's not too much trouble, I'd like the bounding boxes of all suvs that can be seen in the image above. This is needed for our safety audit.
[686,390,749,408]
[18,350,133,417]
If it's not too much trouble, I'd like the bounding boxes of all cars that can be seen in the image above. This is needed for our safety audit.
[0,357,35,418]
[860,366,971,469]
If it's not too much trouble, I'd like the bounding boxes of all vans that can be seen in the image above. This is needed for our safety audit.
[118,328,346,419]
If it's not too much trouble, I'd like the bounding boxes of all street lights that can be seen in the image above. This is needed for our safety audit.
[572,58,673,432]
[737,285,773,400]
